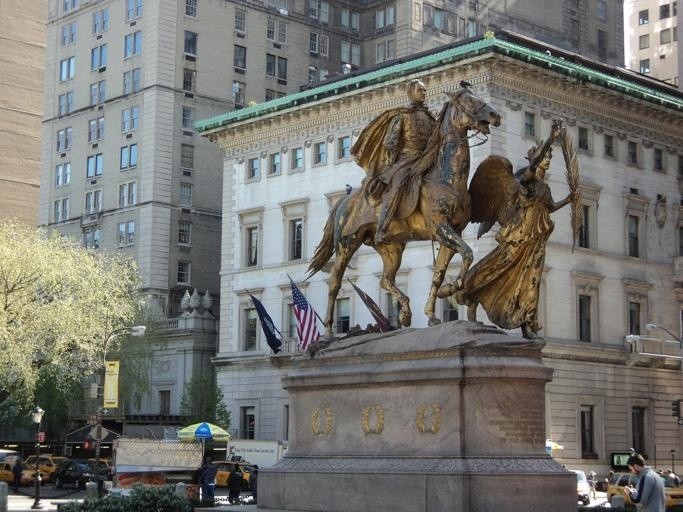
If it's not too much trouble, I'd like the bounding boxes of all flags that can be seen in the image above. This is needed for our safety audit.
[350,284,391,331]
[290,280,320,354]
[250,296,282,354]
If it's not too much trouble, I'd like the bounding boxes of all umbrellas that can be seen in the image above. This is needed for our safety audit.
[178,422,230,457]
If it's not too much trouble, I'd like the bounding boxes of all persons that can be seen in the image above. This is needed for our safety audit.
[198,456,218,503]
[657,468,680,486]
[623,454,666,512]
[226,463,243,505]
[374,78,443,246]
[467,118,581,340]
[249,464,258,500]
[587,470,614,500]
[11,458,23,494]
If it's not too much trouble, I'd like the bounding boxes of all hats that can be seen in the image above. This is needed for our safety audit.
[589,471,596,475]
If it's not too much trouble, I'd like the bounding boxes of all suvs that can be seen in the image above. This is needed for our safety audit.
[561,466,592,503]
[605,471,682,510]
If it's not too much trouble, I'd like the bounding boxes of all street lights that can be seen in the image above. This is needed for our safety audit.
[646,322,682,346]
[96,323,145,504]
[30,404,46,508]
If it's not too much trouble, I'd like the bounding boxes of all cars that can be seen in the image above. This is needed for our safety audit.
[0,444,260,491]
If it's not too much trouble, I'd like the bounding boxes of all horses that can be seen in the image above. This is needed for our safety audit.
[301,80,502,341]
